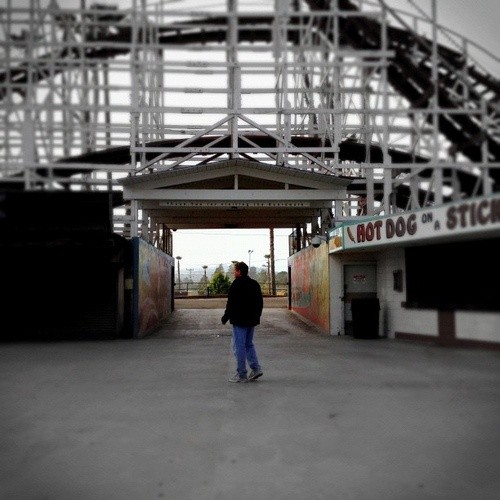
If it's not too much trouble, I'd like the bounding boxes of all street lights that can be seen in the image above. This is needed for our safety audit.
[202,265,207,278]
[247,249,254,273]
[175,256,181,291]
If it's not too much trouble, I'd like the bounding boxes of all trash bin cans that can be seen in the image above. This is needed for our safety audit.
[351,295,380,340]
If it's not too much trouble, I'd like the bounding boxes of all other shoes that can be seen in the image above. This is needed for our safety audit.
[229,373,248,383]
[248,368,264,382]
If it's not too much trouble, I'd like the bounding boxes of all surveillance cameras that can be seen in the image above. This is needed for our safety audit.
[311,237,321,248]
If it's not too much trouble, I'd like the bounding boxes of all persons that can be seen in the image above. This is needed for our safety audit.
[221,262,263,383]
